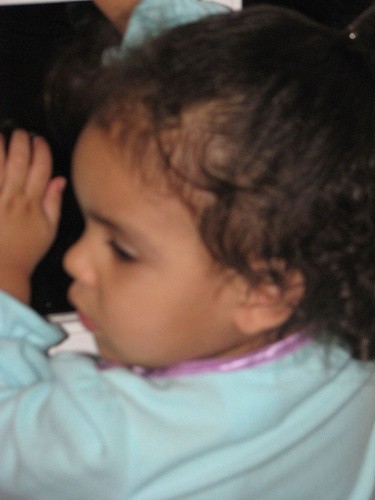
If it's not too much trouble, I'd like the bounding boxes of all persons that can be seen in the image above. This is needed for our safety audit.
[0,9,373,497]
